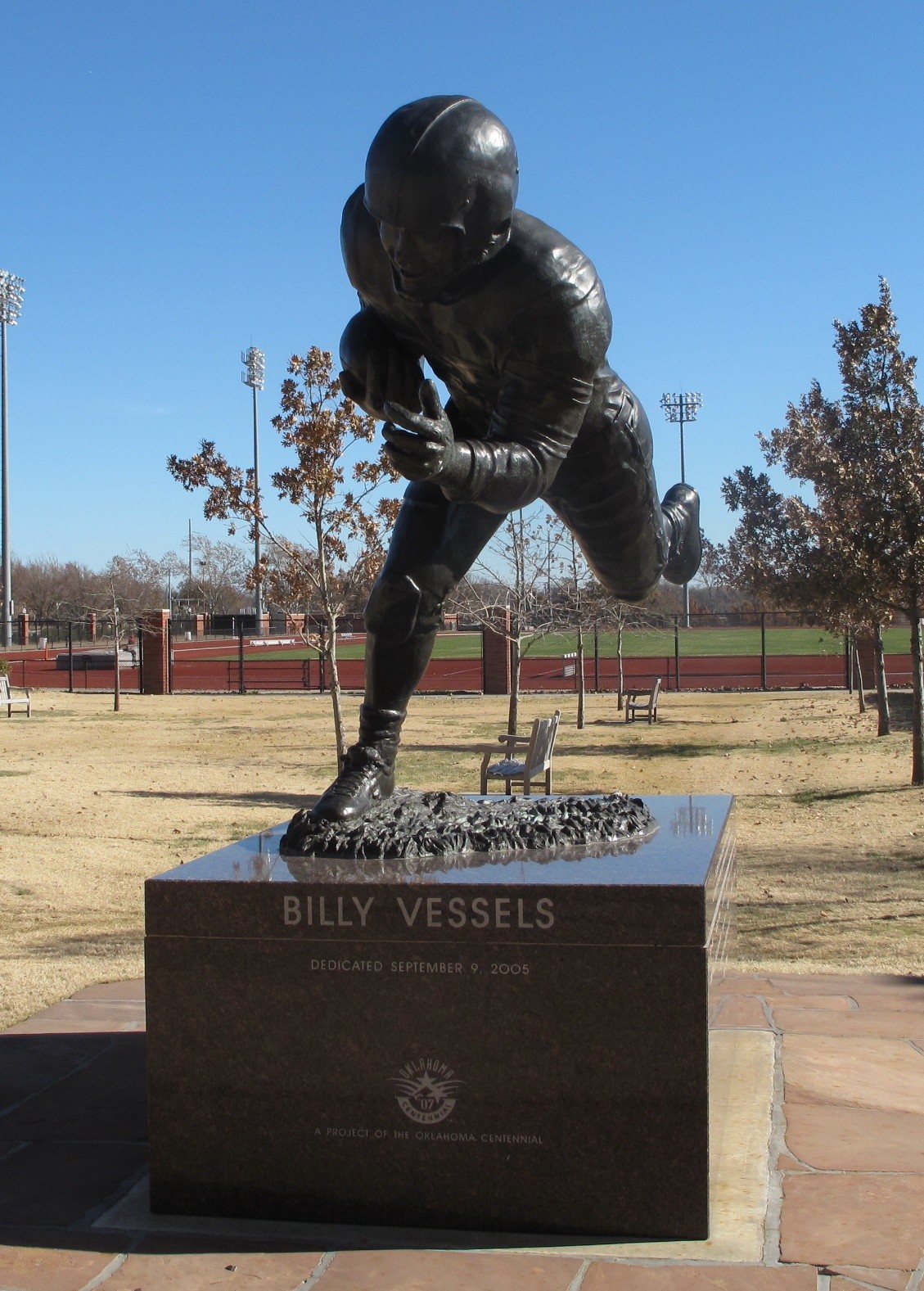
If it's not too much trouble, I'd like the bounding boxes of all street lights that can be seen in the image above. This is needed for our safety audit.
[0,269,26,647]
[659,392,703,629]
[240,346,265,635]
[115,607,119,635]
[188,608,191,632]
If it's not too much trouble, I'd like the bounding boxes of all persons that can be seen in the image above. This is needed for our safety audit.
[313,93,704,822]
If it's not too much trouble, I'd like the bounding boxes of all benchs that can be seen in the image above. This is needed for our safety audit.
[0,673,34,718]
[622,678,661,723]
[476,710,561,795]
